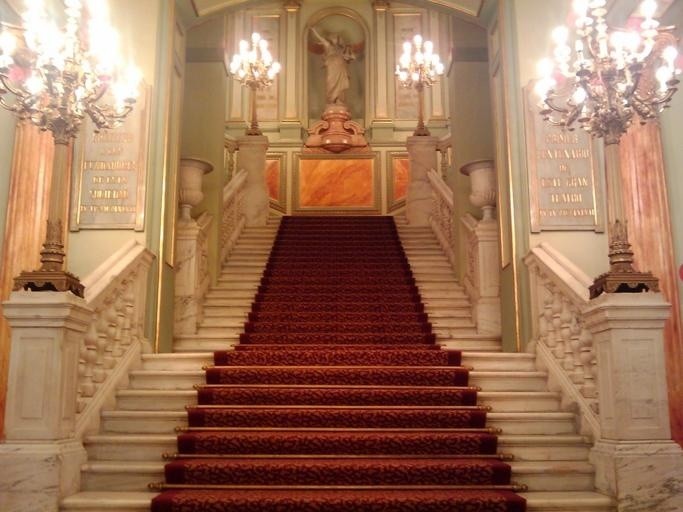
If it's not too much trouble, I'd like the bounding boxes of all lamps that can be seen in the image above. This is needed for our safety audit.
[395,33,444,137]
[229,31,282,137]
[533,1,682,298]
[0,1,142,295]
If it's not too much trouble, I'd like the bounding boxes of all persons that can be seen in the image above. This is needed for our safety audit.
[309,19,357,104]
